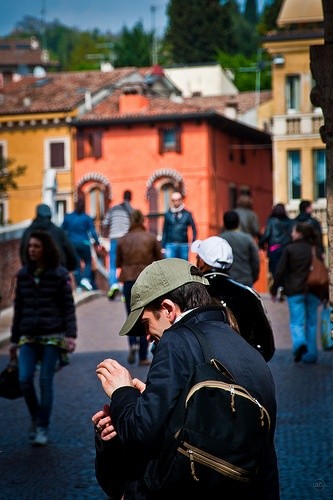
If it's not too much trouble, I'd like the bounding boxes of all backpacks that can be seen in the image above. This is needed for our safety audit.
[129,361,274,500]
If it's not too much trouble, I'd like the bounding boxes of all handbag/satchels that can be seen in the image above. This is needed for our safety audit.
[308,246,332,298]
[1,347,22,400]
[320,303,332,350]
[94,240,108,256]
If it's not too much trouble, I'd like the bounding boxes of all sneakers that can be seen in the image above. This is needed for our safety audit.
[26,428,36,445]
[36,427,48,444]
[81,278,92,293]
[121,295,126,303]
[106,284,118,299]
[76,287,81,293]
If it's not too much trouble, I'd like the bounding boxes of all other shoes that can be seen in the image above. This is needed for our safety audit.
[281,297,287,300]
[277,288,283,302]
[291,343,308,363]
[303,356,319,366]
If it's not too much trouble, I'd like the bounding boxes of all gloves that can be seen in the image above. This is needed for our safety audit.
[63,337,75,352]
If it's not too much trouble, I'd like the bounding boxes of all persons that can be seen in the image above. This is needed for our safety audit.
[19,204,77,288]
[292,200,325,258]
[280,222,330,364]
[265,203,291,302]
[62,201,107,291]
[236,195,260,237]
[107,190,134,301]
[161,192,196,261]
[221,210,261,287]
[117,209,162,364]
[9,231,78,443]
[92,259,280,500]
[191,235,276,362]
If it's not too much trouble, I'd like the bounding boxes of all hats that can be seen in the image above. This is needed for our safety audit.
[118,257,208,336]
[37,204,51,219]
[223,211,239,230]
[191,236,233,271]
